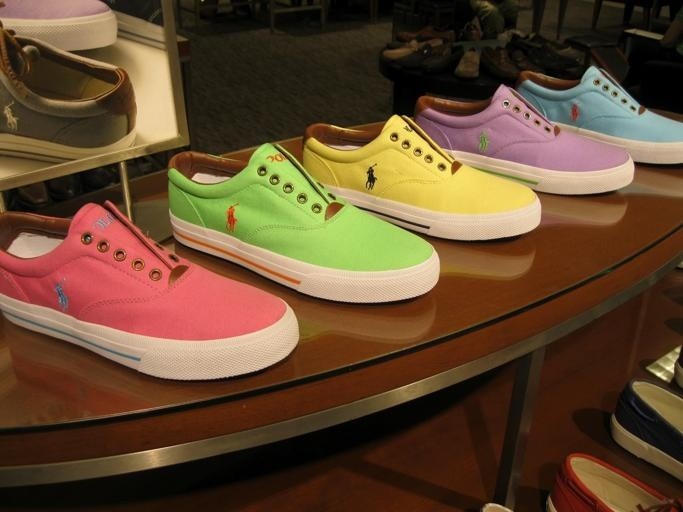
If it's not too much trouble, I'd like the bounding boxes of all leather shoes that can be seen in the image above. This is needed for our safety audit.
[10,156,154,208]
[377,21,584,79]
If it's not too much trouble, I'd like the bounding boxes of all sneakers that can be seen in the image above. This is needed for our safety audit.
[300,113,543,242]
[0,0,118,53]
[610,376,682,480]
[0,200,300,384]
[544,452,682,512]
[413,83,635,197]
[166,139,443,306]
[0,22,137,165]
[516,64,682,166]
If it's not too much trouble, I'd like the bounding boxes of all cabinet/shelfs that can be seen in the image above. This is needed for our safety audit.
[0,113,683,511]
